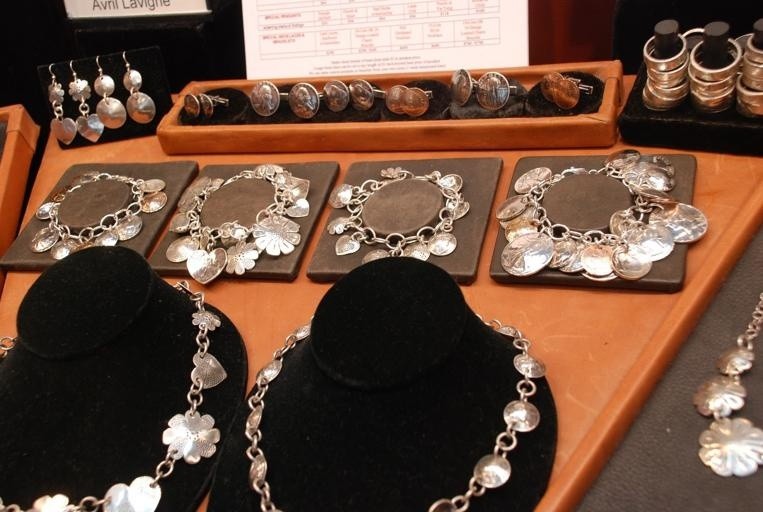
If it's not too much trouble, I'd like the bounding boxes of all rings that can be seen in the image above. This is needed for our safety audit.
[182,66,597,118]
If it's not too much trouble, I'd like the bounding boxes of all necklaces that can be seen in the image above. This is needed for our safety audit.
[0,277,232,512]
[492,148,708,284]
[242,307,545,511]
[325,165,471,266]
[164,161,312,286]
[688,292,762,479]
[27,168,168,263]
